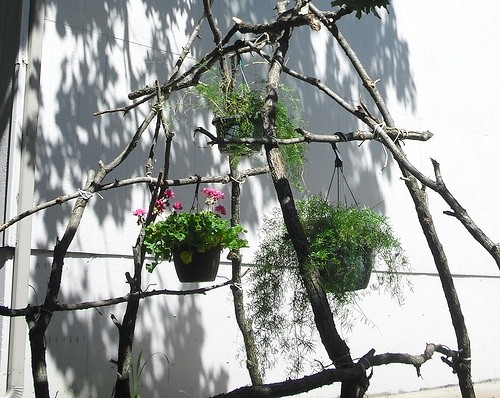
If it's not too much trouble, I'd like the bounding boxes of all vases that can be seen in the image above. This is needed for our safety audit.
[173,242,222,283]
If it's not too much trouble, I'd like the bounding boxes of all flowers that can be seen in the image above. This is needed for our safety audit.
[133,186,250,273]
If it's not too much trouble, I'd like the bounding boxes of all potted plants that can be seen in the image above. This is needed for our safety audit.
[235,132,419,380]
[153,39,311,195]
